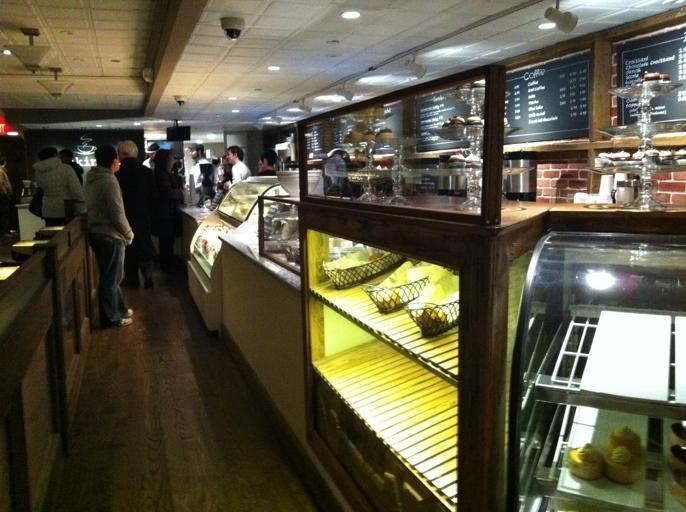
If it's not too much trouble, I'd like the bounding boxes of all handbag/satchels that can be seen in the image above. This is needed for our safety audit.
[29,186,44,217]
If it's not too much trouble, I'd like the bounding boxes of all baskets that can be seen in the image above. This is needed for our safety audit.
[365,260,453,313]
[404,291,460,336]
[323,253,401,290]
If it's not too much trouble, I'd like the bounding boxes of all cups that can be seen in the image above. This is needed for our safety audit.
[598,175,614,204]
[573,192,596,205]
[613,172,629,206]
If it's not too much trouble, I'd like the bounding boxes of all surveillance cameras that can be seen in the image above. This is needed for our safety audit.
[175,96,187,107]
[221,17,244,40]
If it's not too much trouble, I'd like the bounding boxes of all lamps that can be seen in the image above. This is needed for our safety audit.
[4,30,55,74]
[37,67,73,99]
[253,1,579,130]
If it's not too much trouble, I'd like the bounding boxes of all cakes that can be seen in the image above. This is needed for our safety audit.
[668,421,686,507]
[567,423,647,484]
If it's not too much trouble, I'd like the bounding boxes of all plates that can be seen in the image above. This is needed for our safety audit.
[555,405,650,508]
[661,420,686,511]
[577,308,672,405]
[668,311,686,404]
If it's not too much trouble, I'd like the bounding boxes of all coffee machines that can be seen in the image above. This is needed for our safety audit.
[437,154,467,197]
[504,149,539,201]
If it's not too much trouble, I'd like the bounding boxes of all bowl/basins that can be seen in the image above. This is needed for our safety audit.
[278,170,320,198]
[669,421,686,507]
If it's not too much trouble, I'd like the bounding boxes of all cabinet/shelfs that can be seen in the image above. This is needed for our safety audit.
[507,231,685,512]
[218,233,305,446]
[187,179,279,333]
[299,194,548,512]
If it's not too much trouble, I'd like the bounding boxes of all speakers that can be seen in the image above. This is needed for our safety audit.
[167,126,190,142]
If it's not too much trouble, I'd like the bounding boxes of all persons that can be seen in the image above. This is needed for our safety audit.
[116,143,156,289]
[0,156,18,237]
[372,176,395,197]
[83,145,134,329]
[257,150,277,176]
[319,148,359,197]
[29,147,84,227]
[143,144,251,274]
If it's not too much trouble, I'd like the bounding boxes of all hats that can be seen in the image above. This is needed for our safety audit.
[188,144,203,148]
[145,144,159,153]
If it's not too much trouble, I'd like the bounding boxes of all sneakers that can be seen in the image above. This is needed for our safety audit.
[120,309,133,326]
